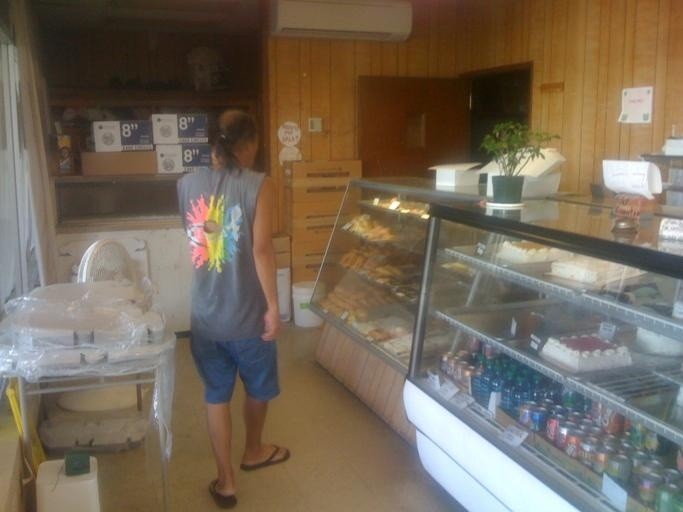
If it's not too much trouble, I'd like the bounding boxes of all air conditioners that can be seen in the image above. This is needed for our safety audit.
[270,0,413,42]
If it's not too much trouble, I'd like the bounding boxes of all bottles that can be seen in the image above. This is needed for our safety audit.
[471,354,561,420]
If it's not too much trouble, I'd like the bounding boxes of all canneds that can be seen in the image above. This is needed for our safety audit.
[440,335,683,512]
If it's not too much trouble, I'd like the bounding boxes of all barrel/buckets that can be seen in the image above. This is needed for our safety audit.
[35,452,101,511]
[292,281,327,329]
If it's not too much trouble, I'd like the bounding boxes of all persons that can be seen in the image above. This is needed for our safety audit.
[176,110,290,508]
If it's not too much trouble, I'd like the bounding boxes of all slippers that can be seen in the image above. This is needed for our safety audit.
[209,480,237,509]
[240,444,290,471]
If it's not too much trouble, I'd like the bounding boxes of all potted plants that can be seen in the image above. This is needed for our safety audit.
[481,123,560,211]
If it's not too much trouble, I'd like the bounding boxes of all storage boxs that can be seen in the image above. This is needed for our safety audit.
[79,113,213,177]
[281,159,363,283]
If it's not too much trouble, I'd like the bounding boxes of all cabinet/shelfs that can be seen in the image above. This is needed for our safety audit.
[0,311,176,512]
[307,172,683,511]
